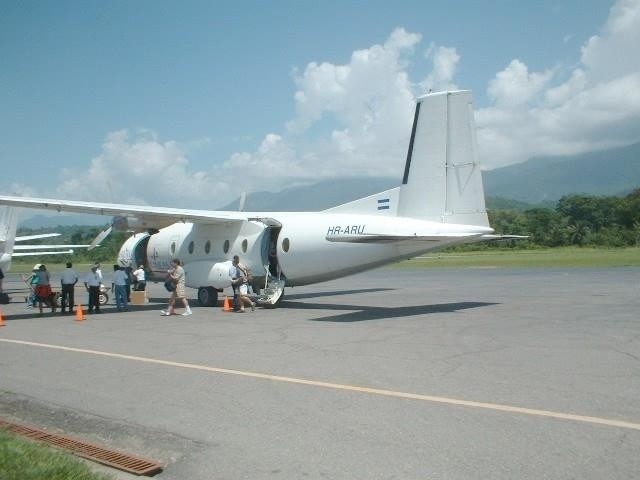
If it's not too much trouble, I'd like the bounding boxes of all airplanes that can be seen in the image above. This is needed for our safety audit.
[0,88,537,309]
[0,192,103,274]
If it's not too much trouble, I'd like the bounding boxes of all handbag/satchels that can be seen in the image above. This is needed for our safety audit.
[164,277,176,292]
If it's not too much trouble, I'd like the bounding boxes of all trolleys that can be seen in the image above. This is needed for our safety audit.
[20,274,69,306]
[82,280,111,305]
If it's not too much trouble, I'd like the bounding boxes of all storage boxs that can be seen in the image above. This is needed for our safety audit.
[130,290,152,305]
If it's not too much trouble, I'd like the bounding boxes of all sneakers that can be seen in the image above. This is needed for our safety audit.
[237,308,245,313]
[182,309,193,316]
[251,302,256,311]
[161,309,170,316]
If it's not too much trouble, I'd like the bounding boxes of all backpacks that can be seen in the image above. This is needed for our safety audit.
[239,266,252,283]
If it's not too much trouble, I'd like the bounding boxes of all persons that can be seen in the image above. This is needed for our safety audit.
[61,262,78,313]
[133,265,146,291]
[84,266,102,313]
[229,256,245,311]
[112,265,129,311]
[268,241,277,279]
[30,265,49,287]
[161,259,192,316]
[0,269,4,296]
[237,263,256,312]
[25,264,41,308]
[95,262,103,283]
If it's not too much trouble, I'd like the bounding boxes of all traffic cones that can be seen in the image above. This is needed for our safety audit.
[0,310,7,327]
[221,295,232,313]
[73,304,85,322]
